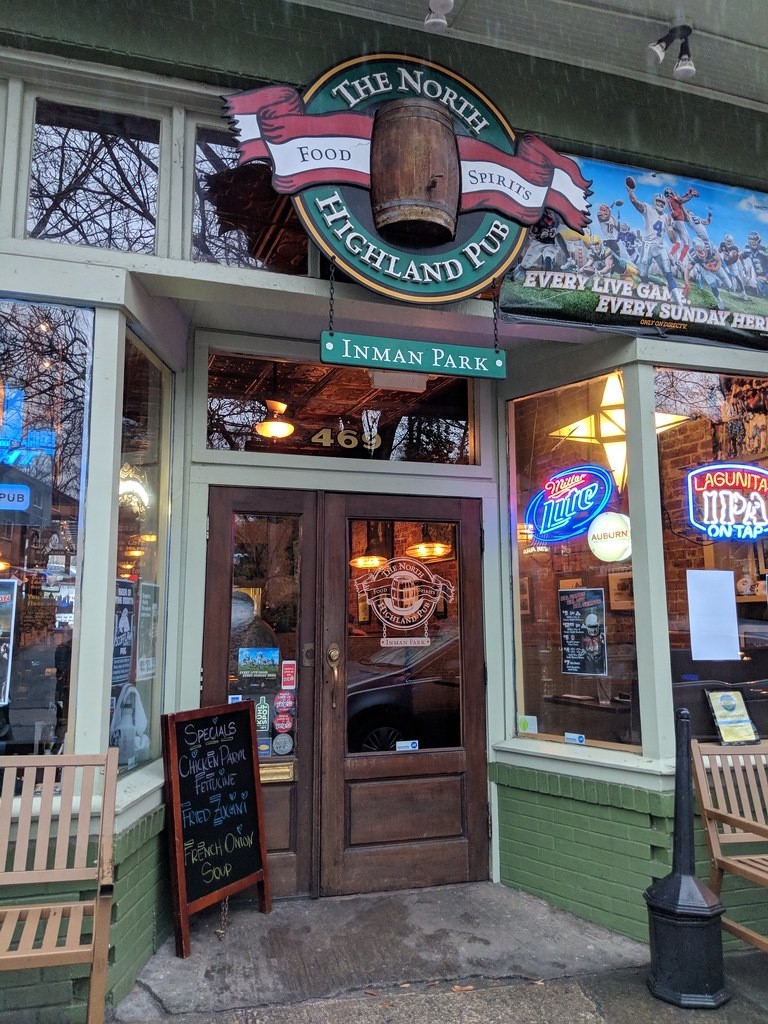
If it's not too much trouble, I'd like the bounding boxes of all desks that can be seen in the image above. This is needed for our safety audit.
[542,696,630,714]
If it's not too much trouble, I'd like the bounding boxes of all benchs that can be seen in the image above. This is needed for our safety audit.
[0,747,120,1024]
[689,738,768,954]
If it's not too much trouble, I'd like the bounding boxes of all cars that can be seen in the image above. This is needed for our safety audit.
[347,623,460,752]
[622,612,768,746]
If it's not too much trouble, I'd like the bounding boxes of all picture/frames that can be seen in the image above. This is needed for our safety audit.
[757,535,768,574]
[519,571,533,621]
[558,576,585,589]
[433,586,448,620]
[400,582,423,624]
[366,520,395,575]
[376,587,390,626]
[356,591,372,625]
[607,571,634,612]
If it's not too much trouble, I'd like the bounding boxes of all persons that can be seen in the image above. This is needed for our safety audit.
[228,590,282,677]
[512,187,768,311]
[347,613,367,637]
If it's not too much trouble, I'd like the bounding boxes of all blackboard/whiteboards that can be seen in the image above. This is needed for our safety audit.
[162,699,269,918]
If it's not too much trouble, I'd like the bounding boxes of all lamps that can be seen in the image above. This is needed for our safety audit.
[405,524,451,559]
[647,17,696,82]
[255,400,295,444]
[549,371,692,504]
[349,521,387,569]
[424,0,454,31]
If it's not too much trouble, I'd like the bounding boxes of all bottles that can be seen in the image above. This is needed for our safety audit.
[255,696,269,731]
[113,694,135,765]
